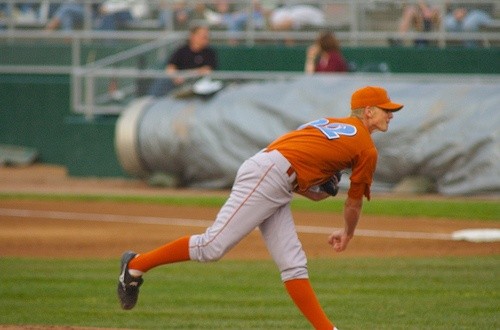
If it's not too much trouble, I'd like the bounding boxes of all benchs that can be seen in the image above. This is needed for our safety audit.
[210,46,500,74]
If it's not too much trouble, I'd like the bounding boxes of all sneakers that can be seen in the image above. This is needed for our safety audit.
[117,251,143,310]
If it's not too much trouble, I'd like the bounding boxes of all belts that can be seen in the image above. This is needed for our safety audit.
[262,149,300,193]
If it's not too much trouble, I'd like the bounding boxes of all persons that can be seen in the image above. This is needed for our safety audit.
[304,31,347,72]
[400,0,441,46]
[0,0,325,32]
[441,0,500,47]
[147,20,216,96]
[119,86,403,330]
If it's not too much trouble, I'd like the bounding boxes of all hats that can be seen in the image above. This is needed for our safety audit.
[351,86,404,112]
